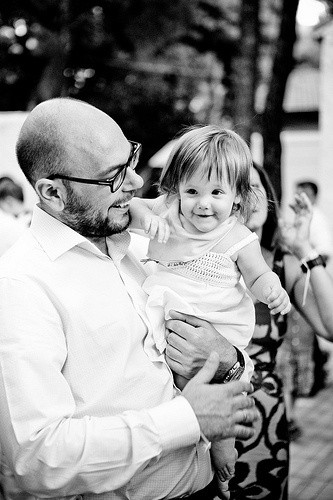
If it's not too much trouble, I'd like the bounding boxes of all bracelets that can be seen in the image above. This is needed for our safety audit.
[300,255,326,274]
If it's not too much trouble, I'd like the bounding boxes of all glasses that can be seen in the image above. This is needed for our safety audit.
[46,140,143,193]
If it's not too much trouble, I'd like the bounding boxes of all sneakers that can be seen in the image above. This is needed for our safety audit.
[287,418,301,441]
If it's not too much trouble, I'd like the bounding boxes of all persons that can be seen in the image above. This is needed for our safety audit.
[0,174,333,443]
[0,97,260,500]
[129,126,291,500]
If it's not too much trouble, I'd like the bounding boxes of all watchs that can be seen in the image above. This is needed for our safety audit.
[222,346,245,384]
